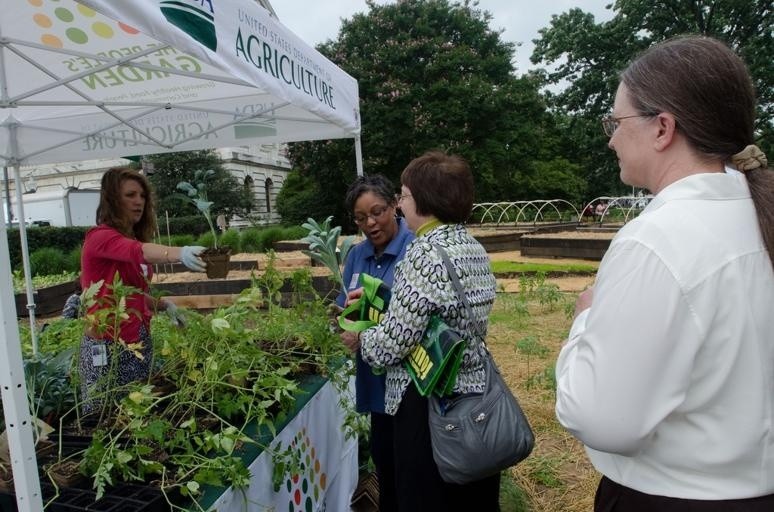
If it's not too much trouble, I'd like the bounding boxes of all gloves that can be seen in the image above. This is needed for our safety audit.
[181,246,207,273]
[165,301,188,328]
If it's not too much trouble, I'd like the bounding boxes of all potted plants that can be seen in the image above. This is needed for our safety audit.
[0,169,371,512]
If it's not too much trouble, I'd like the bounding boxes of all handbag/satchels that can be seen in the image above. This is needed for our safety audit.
[339,271,464,399]
[428,346,535,489]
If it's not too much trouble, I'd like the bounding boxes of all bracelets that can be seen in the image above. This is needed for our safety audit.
[357,330,363,343]
[163,244,172,265]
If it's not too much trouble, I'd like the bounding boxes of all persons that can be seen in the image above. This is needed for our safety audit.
[61,278,84,320]
[74,166,210,420]
[336,147,506,512]
[324,174,423,512]
[583,201,594,217]
[547,30,774,512]
[595,198,610,226]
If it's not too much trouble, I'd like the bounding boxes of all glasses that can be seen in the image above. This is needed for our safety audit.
[352,199,393,225]
[602,112,659,137]
[393,194,412,205]
